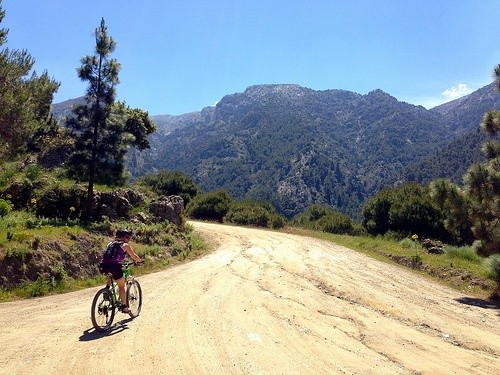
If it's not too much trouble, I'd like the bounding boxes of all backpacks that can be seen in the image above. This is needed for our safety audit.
[103,241,121,259]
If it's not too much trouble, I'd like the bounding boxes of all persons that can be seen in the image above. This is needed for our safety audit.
[100,230,142,313]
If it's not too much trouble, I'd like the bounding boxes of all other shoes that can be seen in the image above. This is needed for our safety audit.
[103,293,109,299]
[122,305,131,312]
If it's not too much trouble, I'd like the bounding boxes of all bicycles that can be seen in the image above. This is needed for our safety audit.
[91,260,146,334]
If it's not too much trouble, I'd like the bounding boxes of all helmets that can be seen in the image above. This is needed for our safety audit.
[116,229,132,237]
